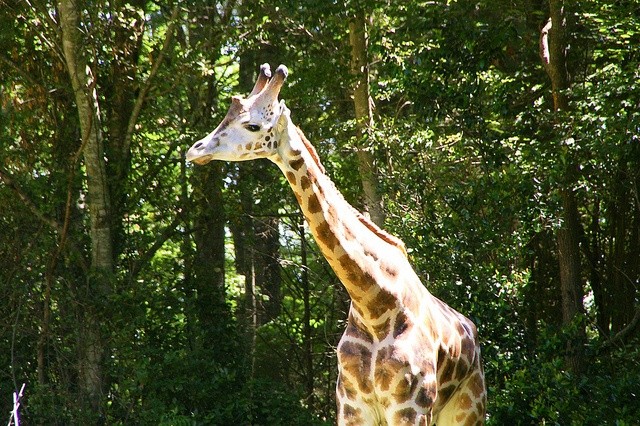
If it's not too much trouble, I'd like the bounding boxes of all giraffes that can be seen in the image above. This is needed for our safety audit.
[186,62,488,426]
